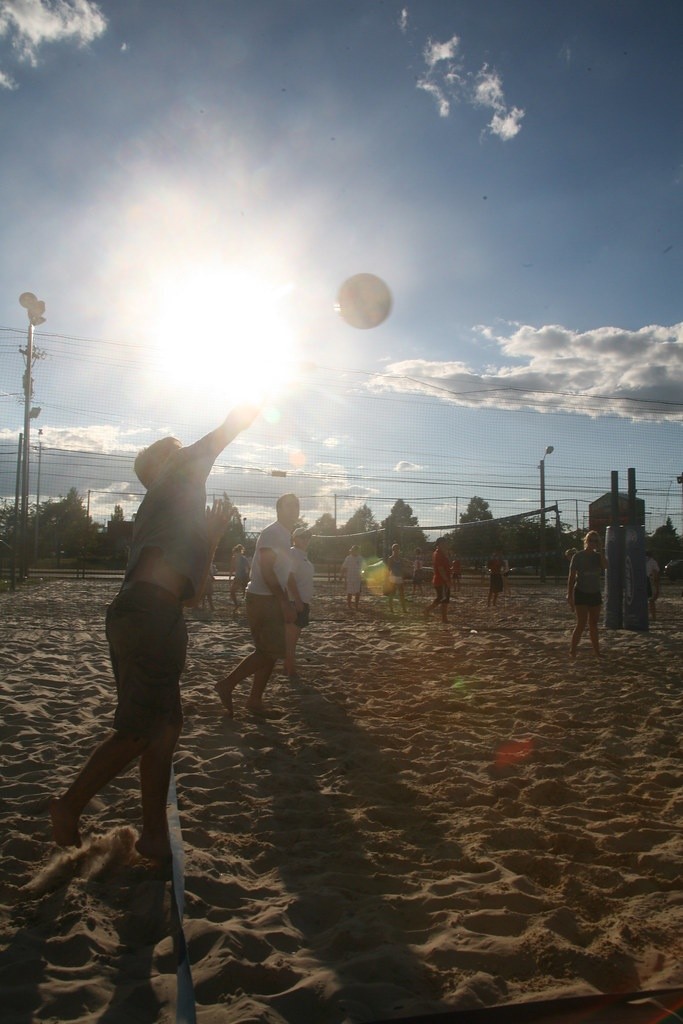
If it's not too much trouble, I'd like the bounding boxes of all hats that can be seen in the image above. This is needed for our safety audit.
[292,526,313,540]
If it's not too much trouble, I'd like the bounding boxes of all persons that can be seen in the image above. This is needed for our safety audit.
[46,401,662,863]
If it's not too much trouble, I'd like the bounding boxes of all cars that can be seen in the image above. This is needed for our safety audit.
[663,559,683,580]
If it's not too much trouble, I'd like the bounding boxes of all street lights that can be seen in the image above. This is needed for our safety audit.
[539,446,556,581]
[19,291,45,570]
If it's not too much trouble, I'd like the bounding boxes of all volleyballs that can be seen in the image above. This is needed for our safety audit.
[336,272,392,330]
